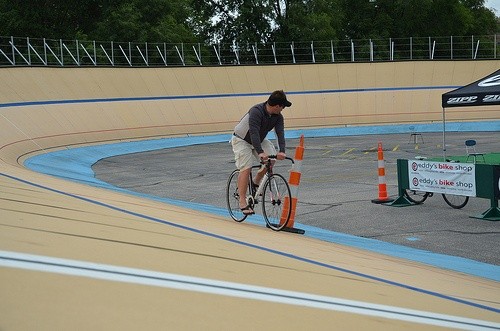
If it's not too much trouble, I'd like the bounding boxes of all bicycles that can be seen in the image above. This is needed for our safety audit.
[225,154,295,232]
[403,153,469,209]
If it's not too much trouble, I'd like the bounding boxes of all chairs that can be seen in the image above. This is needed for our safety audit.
[408,126,423,145]
[465,139,487,165]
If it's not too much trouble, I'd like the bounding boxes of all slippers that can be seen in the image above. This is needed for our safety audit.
[241,205,254,214]
[254,184,263,196]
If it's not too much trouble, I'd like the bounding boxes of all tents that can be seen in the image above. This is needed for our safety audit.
[442,68,500,163]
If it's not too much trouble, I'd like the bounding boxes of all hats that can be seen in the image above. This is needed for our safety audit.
[267,97,291,107]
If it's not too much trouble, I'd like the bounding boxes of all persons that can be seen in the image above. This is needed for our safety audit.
[229,90,292,215]
